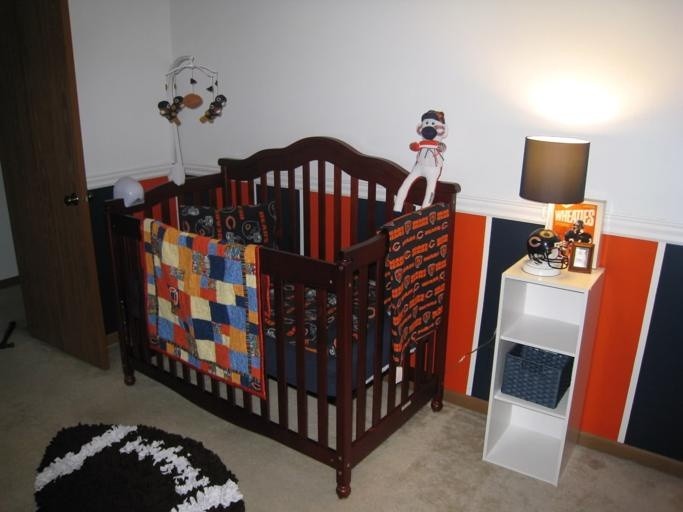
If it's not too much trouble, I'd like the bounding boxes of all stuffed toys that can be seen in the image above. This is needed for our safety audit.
[158,94,227,125]
[390,110,447,214]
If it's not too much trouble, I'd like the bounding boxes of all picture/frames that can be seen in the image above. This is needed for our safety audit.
[545,197,608,270]
[568,240,595,274]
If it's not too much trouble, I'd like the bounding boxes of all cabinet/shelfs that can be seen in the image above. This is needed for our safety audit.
[482,255,606,489]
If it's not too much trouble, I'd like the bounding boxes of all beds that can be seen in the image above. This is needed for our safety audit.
[103,136,461,501]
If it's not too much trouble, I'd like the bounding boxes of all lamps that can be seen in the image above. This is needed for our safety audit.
[519,135,592,277]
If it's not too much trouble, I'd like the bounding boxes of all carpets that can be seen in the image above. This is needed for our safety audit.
[33,418,246,512]
